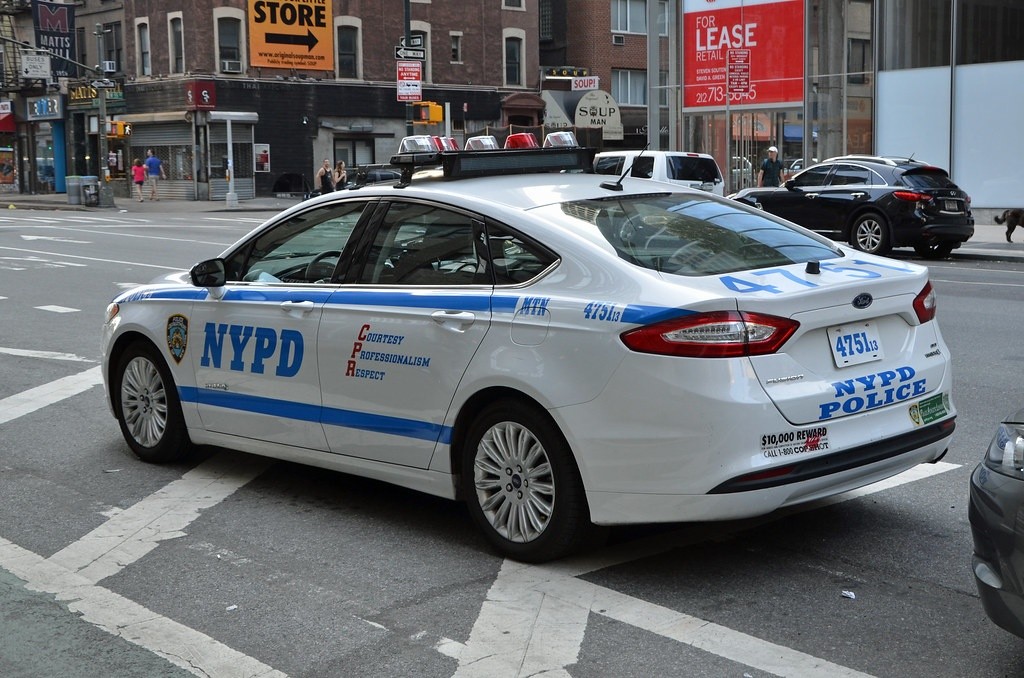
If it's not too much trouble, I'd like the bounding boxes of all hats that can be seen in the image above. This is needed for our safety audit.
[767,146,778,154]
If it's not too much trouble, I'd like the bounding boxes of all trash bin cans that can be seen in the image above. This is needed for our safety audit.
[79,176,98,205]
[65,176,81,205]
[81,185,99,207]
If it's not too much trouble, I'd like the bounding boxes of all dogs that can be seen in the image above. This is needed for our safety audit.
[994,208,1024,242]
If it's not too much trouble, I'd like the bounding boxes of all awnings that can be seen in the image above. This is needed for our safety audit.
[732,114,776,141]
[621,113,669,136]
[784,125,818,142]
[0,114,16,132]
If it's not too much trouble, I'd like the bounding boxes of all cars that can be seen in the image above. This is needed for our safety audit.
[823,153,930,171]
[731,156,757,194]
[969,409,1024,637]
[101,132,959,565]
[779,158,818,182]
[730,159,975,261]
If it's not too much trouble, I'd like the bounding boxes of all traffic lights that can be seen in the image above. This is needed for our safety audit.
[420,104,443,123]
[117,122,132,137]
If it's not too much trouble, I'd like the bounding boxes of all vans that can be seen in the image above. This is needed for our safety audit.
[562,149,725,198]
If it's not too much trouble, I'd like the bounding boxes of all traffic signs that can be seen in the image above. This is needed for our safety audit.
[22,55,50,78]
[400,35,423,47]
[246,0,335,70]
[395,46,426,61]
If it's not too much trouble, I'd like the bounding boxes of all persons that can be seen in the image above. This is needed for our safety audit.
[757,146,785,188]
[334,160,347,191]
[316,158,334,195]
[143,148,167,201]
[132,159,147,202]
